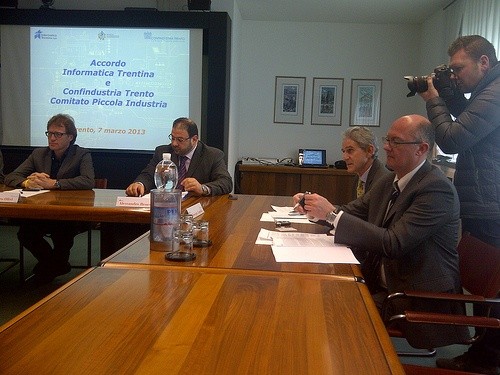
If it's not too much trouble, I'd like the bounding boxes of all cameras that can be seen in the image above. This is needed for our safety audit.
[404,64,453,100]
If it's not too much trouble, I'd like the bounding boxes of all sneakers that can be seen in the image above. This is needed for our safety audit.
[436,356,500,375]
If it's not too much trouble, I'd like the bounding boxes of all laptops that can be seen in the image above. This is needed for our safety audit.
[299,149,329,169]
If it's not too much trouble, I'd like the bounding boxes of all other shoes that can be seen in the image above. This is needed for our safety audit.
[30,259,70,287]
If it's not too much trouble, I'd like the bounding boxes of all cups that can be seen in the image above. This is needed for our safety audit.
[149,188,182,252]
[170,213,209,258]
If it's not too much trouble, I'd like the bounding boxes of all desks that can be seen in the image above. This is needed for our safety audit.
[0,188,405,375]
[237,164,358,203]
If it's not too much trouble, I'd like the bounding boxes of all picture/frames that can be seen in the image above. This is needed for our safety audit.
[349,77,382,128]
[311,78,344,126]
[273,74,307,125]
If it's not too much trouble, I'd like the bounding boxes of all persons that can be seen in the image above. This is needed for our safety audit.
[418,35,500,371]
[125,117,233,197]
[3,113,95,283]
[341,126,391,200]
[293,114,472,349]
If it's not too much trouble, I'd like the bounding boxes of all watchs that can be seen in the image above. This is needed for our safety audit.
[202,185,208,196]
[326,208,341,225]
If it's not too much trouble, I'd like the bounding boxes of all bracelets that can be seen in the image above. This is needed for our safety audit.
[54,180,61,190]
[25,179,28,187]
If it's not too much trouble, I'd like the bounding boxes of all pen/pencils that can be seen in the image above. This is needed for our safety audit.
[19,190,23,194]
[139,193,141,197]
[294,196,304,209]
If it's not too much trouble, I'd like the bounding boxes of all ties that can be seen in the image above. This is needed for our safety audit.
[179,156,188,191]
[370,180,400,270]
[356,180,364,198]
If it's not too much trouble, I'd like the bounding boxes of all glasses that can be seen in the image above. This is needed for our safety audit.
[45,132,69,138]
[168,133,193,142]
[381,136,423,148]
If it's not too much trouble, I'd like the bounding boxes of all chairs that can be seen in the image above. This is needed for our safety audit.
[18,177,109,284]
[383,230,500,357]
[387,309,500,375]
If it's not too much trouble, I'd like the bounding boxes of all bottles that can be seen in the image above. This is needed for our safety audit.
[154,153,178,243]
